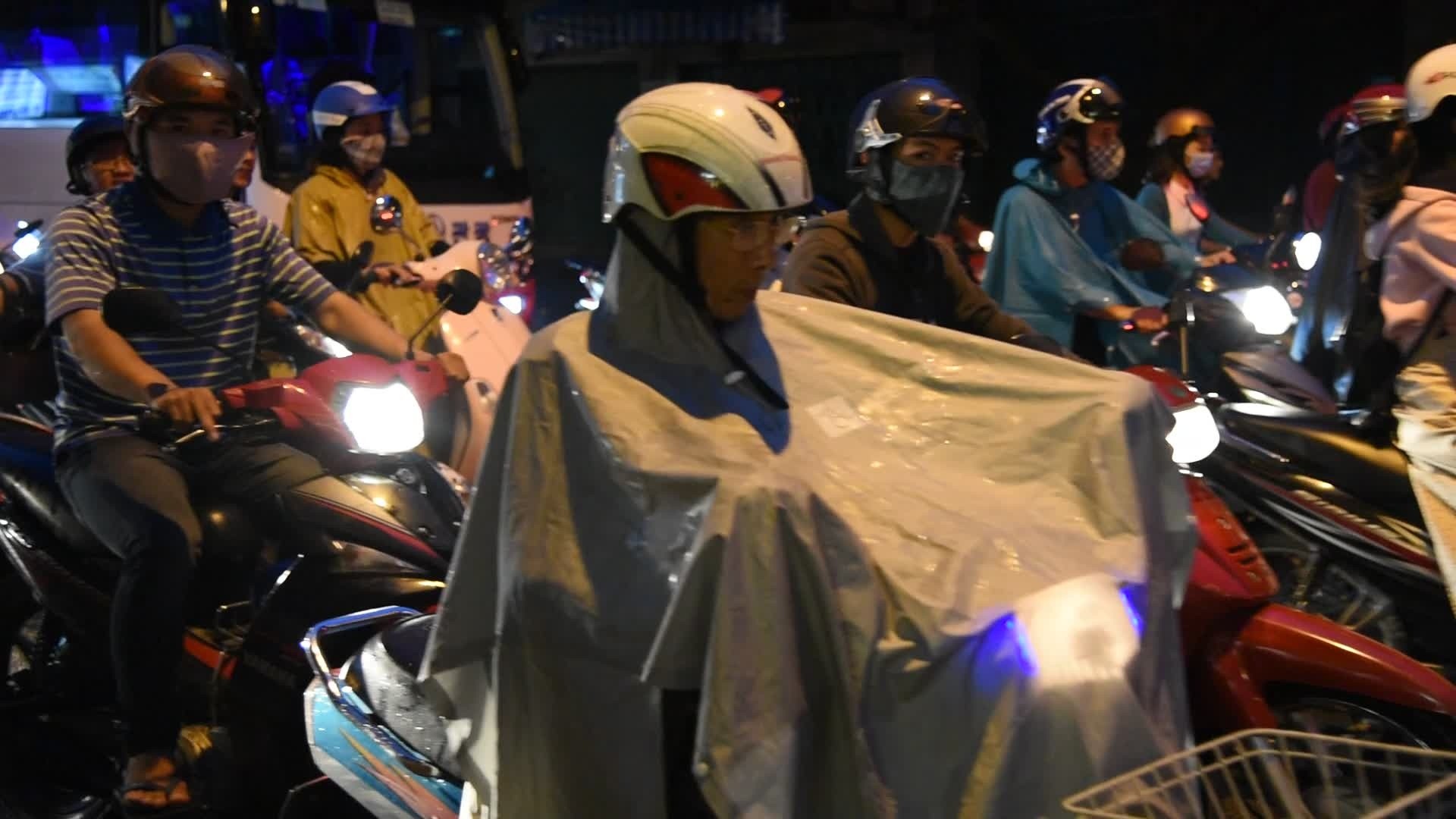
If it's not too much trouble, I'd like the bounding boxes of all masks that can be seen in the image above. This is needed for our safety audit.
[1067,145,1126,181]
[1183,152,1213,178]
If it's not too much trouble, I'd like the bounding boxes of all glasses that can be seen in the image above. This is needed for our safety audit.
[701,213,811,250]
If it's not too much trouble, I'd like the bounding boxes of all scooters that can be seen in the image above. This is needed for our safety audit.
[0,214,1456,819]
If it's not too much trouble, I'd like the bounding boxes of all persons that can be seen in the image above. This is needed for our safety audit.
[1299,42,1456,611]
[1129,107,1263,264]
[0,44,453,819]
[780,75,1086,362]
[421,82,1198,819]
[983,79,1203,360]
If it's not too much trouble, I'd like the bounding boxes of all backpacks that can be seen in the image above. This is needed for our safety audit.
[1328,197,1456,451]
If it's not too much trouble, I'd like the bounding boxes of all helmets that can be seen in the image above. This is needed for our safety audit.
[1037,79,1127,147]
[66,114,129,195]
[853,79,979,155]
[121,46,263,137]
[1153,109,1214,147]
[601,80,816,224]
[1339,85,1410,135]
[1406,43,1456,123]
[1318,109,1345,141]
[312,80,397,161]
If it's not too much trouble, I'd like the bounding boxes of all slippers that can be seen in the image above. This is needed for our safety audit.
[115,772,194,818]
